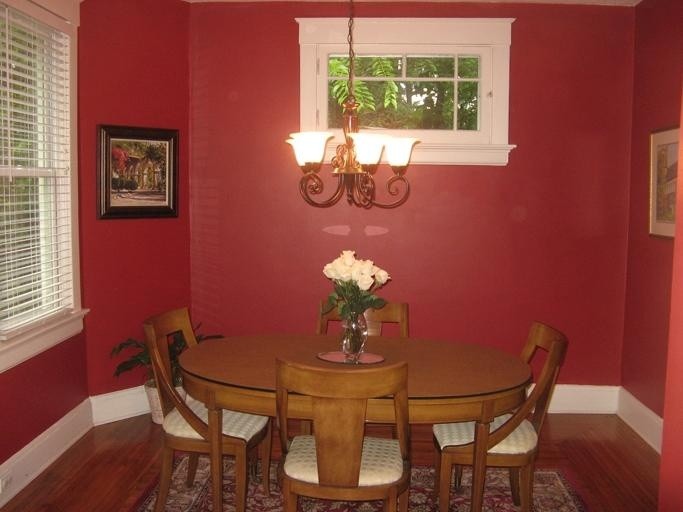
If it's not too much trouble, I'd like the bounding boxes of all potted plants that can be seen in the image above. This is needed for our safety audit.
[109,321,225,424]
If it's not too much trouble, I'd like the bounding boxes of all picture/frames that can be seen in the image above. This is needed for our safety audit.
[647,124,681,240]
[96,124,179,219]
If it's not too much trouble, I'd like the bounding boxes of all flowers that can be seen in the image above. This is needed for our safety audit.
[319,249,393,354]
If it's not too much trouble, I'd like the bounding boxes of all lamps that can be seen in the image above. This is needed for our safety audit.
[285,0,421,209]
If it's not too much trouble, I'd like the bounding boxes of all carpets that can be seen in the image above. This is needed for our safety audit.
[138,450,590,512]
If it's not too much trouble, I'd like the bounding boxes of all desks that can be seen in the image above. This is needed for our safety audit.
[176,332,533,512]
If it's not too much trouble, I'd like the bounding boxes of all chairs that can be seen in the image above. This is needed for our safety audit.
[272,356,413,512]
[143,307,272,512]
[432,319,568,512]
[318,300,409,339]
